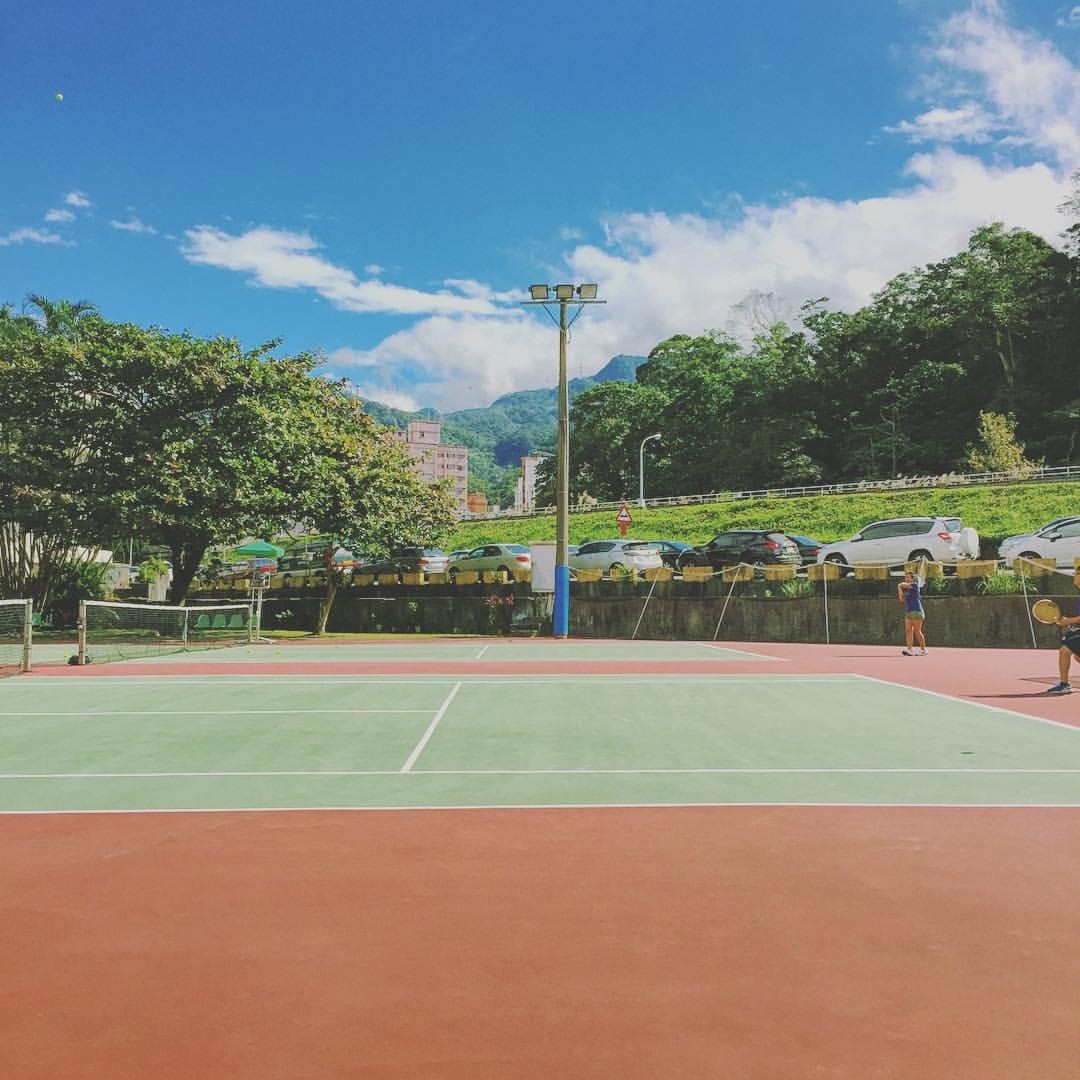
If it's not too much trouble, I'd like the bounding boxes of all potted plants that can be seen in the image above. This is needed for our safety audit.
[138,558,169,601]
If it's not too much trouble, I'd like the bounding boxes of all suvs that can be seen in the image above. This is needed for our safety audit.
[816,515,981,578]
[674,529,802,575]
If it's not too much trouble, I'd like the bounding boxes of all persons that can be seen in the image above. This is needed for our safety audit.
[1048,565,1080,694]
[898,570,928,656]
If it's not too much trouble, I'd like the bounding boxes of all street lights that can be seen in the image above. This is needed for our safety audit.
[638,433,664,507]
[519,282,609,639]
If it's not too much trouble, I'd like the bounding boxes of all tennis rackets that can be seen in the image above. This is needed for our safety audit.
[1031,599,1062,625]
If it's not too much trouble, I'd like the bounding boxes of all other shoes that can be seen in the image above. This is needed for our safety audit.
[903,648,914,656]
[915,648,928,656]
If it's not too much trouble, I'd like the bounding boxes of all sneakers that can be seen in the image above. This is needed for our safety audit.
[1048,681,1073,694]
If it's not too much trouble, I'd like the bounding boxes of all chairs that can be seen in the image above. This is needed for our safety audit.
[31,612,41,632]
[192,614,257,639]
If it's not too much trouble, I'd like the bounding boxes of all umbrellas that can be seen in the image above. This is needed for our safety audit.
[236,540,285,558]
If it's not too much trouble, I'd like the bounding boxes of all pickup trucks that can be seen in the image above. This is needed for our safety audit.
[270,558,330,588]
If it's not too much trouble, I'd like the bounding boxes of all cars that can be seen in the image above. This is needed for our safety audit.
[787,534,824,570]
[350,543,534,584]
[998,515,1080,570]
[246,557,278,574]
[564,539,691,582]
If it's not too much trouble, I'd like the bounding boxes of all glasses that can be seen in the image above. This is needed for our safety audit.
[1072,573,1080,576]
